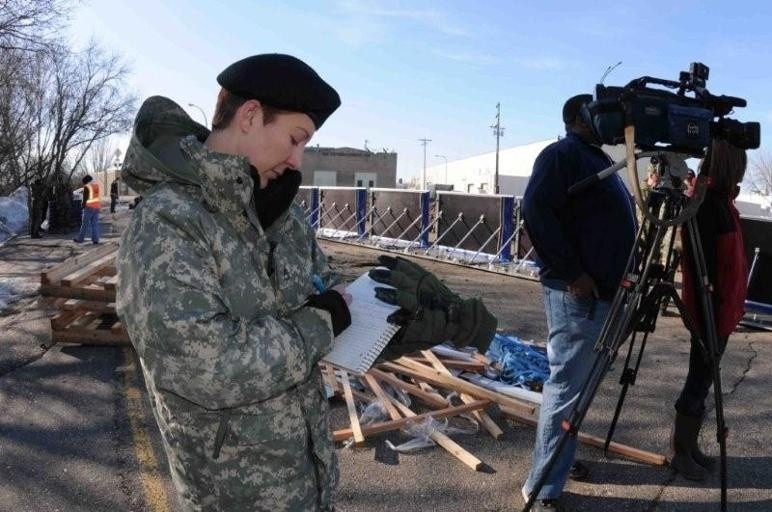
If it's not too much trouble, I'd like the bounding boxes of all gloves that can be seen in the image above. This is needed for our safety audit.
[368,253,498,354]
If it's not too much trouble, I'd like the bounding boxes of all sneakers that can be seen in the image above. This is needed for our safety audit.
[570,462,587,482]
[521,485,560,512]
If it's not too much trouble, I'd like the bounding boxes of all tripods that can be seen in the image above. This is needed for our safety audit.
[519,188,730,512]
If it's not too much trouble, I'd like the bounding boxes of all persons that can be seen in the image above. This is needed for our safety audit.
[116,51,355,512]
[672,166,699,253]
[71,174,101,245]
[635,161,665,266]
[667,140,750,481]
[29,178,49,240]
[109,176,120,214]
[520,91,642,510]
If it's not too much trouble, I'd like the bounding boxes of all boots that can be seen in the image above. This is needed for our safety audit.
[672,412,716,481]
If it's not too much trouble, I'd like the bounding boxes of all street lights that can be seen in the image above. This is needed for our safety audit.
[190,104,209,129]
[434,155,449,186]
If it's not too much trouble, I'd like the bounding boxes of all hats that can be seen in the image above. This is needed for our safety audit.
[216,54,341,130]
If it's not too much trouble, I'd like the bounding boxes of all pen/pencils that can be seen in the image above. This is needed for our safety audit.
[312,274,327,294]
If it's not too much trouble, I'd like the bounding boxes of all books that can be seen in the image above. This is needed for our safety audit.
[316,261,408,376]
